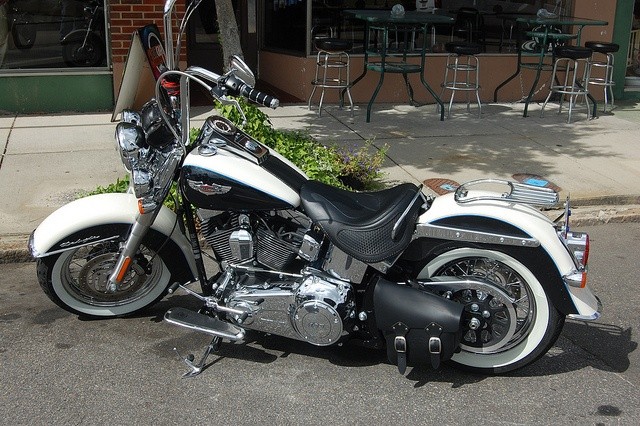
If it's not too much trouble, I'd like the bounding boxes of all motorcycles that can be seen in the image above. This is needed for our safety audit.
[28,0,603,379]
[59,5,105,67]
[2,1,36,49]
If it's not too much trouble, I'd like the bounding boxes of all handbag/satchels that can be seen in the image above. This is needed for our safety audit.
[372,277,465,375]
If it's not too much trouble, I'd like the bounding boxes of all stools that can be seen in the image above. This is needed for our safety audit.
[540,46,592,123]
[308,39,356,116]
[572,41,619,110]
[437,41,483,119]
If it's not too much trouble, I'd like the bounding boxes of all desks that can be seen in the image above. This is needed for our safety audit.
[342,11,462,122]
[494,12,608,119]
